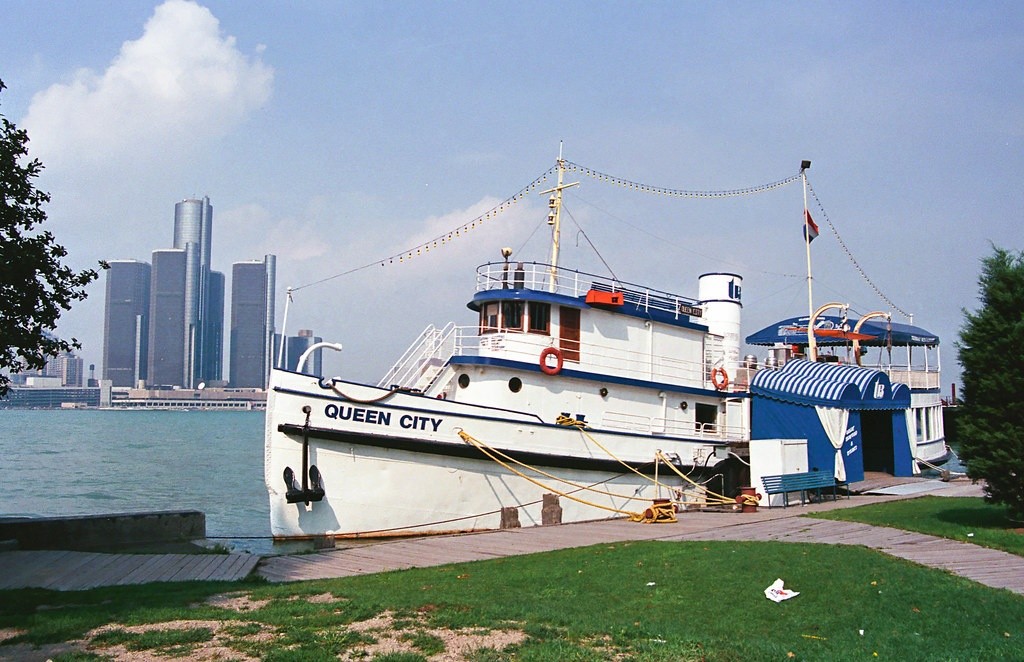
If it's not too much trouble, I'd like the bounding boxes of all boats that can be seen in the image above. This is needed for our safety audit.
[263,136,955,531]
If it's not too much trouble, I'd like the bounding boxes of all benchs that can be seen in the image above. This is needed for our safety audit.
[761,470,849,509]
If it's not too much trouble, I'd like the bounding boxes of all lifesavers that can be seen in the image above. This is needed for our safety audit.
[711,367,728,390]
[539,347,563,375]
